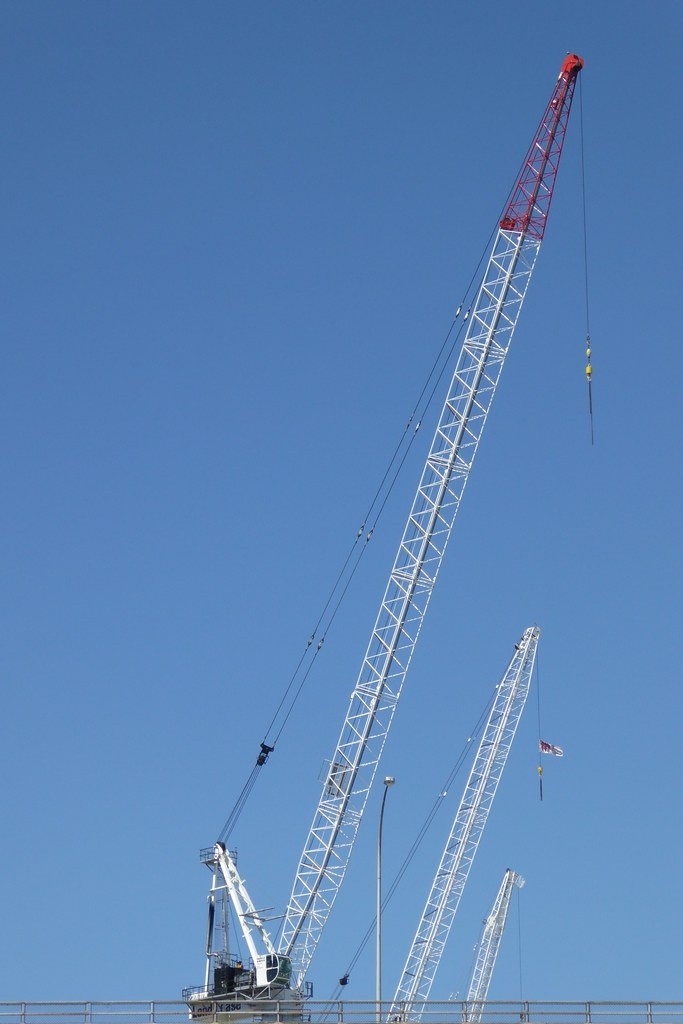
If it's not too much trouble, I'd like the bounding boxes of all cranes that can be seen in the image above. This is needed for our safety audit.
[179,51,594,1024]
[389,626,538,1024]
[458,868,526,1024]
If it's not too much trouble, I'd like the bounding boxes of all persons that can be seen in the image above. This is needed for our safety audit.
[236,961,243,968]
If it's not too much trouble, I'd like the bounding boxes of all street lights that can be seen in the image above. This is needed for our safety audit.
[375,776,396,1024]
